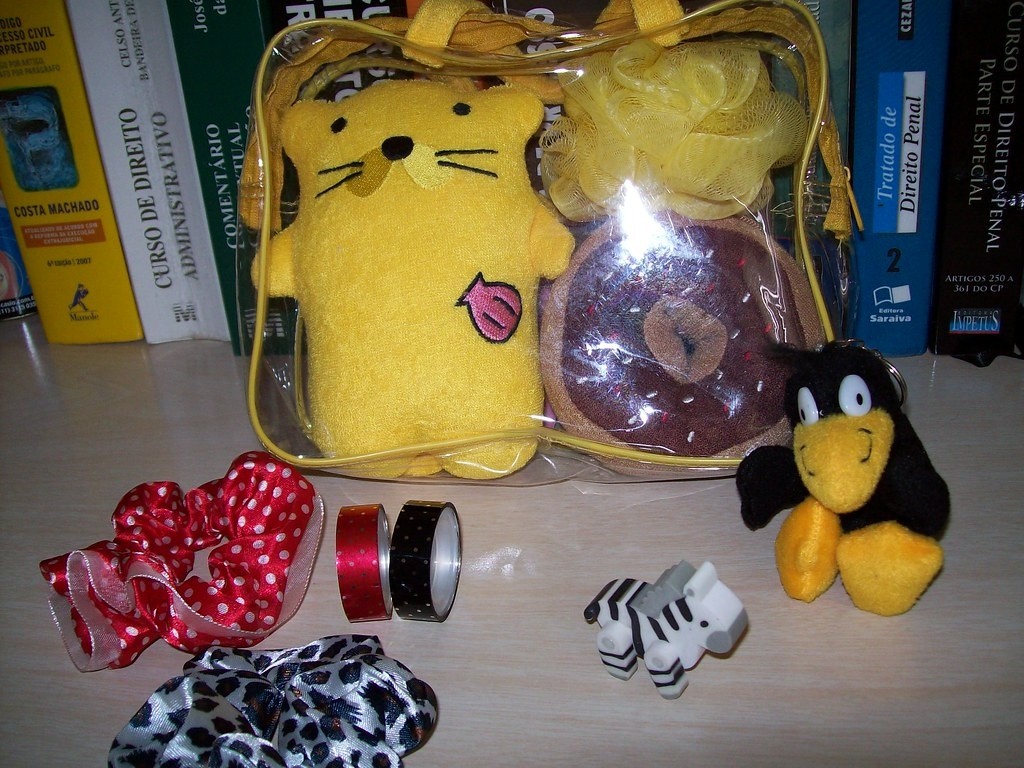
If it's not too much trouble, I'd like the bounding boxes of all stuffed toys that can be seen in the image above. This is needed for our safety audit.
[251,81,568,478]
[736,341,951,613]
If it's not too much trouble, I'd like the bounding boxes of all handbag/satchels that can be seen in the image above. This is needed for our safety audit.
[236,2,864,488]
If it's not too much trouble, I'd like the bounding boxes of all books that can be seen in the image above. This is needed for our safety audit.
[0,0,1024,369]
